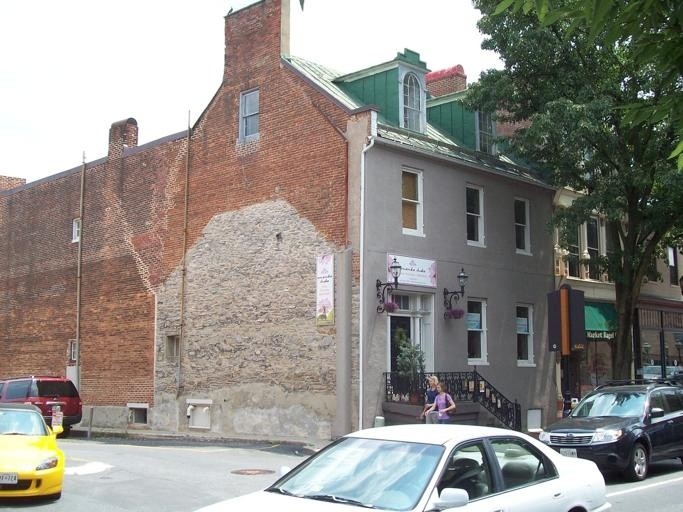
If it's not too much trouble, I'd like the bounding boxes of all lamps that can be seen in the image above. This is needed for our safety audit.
[376,256,401,313]
[442,267,468,321]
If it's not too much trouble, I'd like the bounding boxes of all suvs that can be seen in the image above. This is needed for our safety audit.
[0,375,84,438]
[539,380,682,480]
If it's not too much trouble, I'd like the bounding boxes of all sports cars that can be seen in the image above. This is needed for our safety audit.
[0,402,65,504]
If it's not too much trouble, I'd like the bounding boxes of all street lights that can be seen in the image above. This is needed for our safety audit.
[643,342,650,364]
[664,340,668,365]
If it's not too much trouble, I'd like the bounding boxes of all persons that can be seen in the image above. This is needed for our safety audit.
[424,382,456,424]
[423,376,439,424]
[564,401,582,418]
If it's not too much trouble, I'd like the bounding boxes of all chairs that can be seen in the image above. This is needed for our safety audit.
[500,461,533,489]
[439,458,488,500]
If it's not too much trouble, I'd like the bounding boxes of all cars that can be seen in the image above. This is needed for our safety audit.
[188,422,614,512]
[642,364,681,383]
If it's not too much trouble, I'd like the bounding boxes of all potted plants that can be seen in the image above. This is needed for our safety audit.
[395,338,426,403]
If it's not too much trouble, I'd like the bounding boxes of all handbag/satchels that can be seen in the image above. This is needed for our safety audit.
[446,401,456,416]
